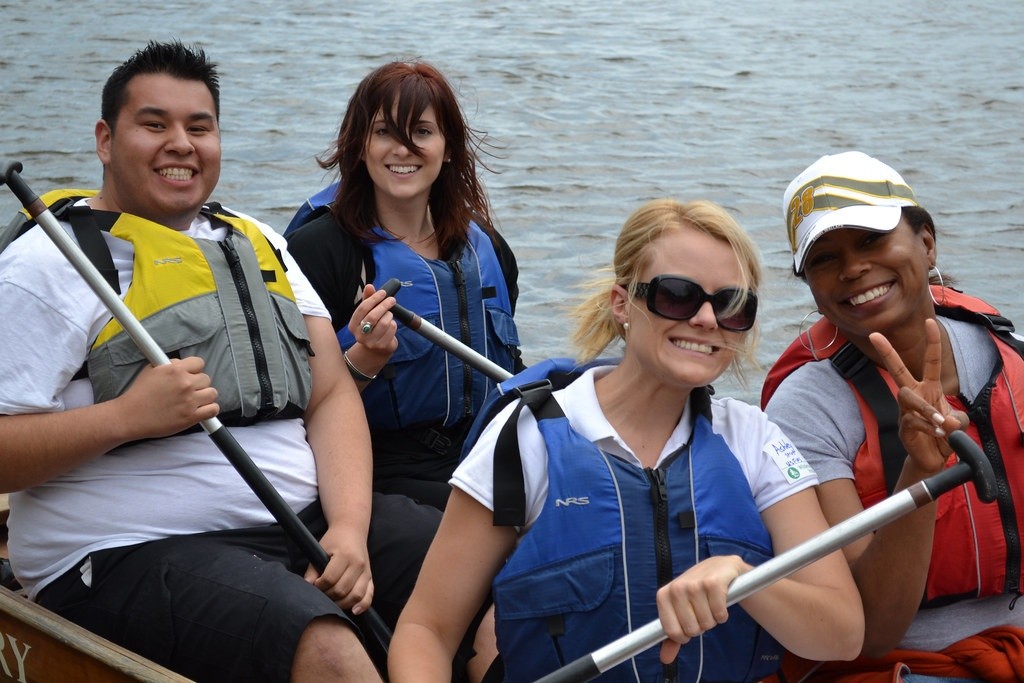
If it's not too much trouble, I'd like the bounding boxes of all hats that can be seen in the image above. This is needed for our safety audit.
[780,152,919,274]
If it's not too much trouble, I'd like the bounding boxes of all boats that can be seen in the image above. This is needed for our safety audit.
[0,489,200,683]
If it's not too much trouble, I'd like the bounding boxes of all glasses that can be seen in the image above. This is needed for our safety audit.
[627,276,760,332]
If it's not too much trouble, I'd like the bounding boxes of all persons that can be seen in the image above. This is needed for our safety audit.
[762,150,1024,683]
[0,39,498,683]
[284,60,529,683]
[386,201,865,683]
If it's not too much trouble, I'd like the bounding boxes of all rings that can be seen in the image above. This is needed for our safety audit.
[360,320,373,335]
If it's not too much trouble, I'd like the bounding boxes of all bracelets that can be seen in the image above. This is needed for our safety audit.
[343,350,377,382]
